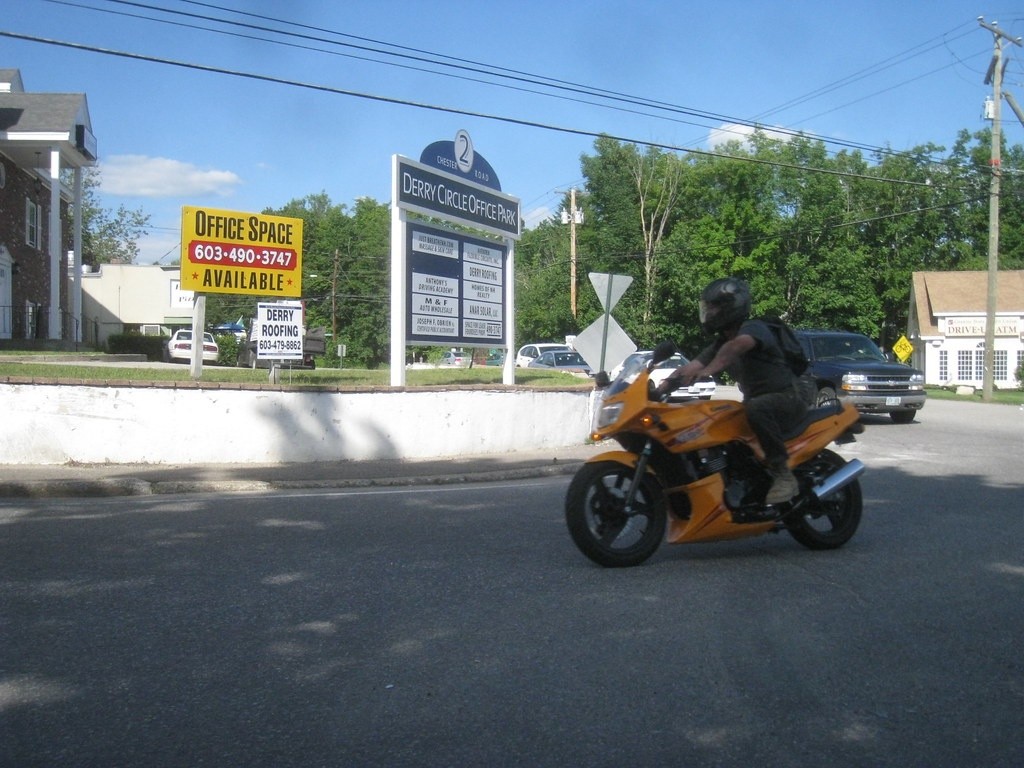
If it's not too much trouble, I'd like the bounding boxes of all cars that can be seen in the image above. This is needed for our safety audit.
[610,350,717,402]
[528,350,597,379]
[168,328,219,366]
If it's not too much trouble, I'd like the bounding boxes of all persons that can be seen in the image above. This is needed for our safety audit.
[650,277,810,503]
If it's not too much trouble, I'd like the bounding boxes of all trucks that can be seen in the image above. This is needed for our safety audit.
[236,329,326,370]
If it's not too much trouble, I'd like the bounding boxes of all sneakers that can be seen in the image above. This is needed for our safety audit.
[767,476,799,504]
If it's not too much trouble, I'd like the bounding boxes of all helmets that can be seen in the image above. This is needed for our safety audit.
[701,278,750,336]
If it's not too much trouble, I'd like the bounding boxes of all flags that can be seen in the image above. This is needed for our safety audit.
[232,317,247,338]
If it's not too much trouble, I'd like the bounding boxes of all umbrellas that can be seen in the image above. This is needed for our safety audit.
[212,321,247,336]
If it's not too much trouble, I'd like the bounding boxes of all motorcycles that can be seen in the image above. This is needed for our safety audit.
[565,342,867,569]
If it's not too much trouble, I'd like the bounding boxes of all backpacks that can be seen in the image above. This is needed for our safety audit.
[737,314,809,379]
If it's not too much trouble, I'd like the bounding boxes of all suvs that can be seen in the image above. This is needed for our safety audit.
[792,328,927,425]
[516,343,572,370]
